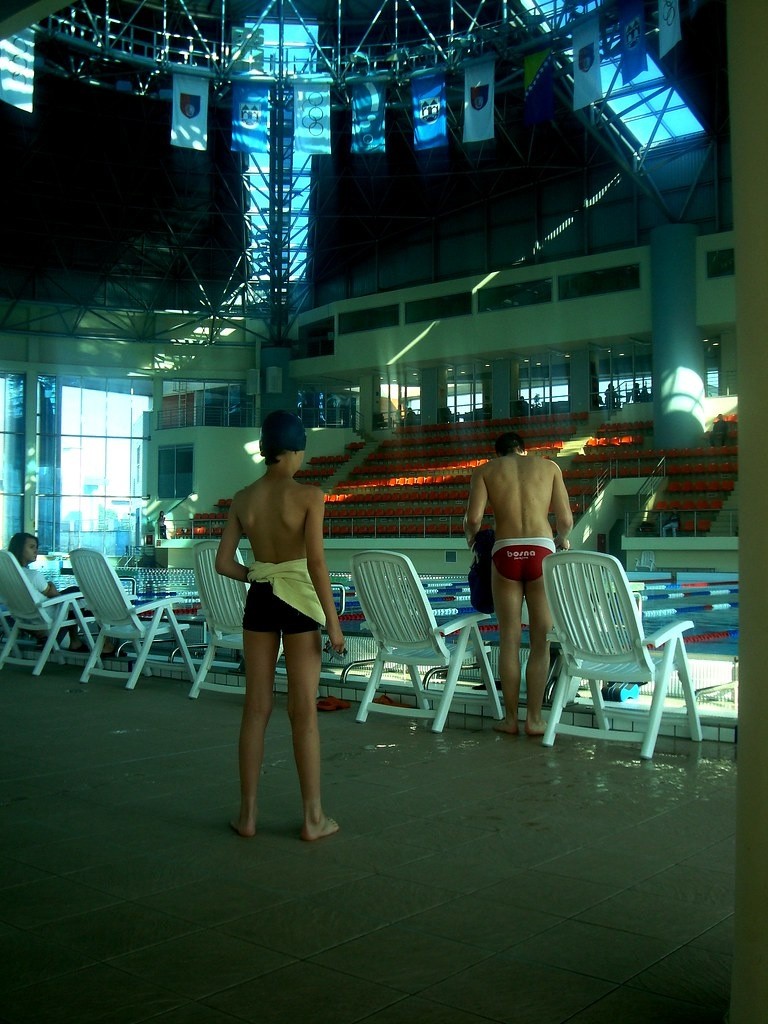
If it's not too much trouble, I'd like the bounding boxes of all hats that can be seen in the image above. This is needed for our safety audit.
[257,409,307,455]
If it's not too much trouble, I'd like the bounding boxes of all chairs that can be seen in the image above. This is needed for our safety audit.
[70,548,200,691]
[188,540,282,710]
[169,405,745,533]
[347,548,503,734]
[0,549,106,677]
[534,548,708,760]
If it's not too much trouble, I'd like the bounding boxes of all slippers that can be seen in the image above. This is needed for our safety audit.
[317,696,351,711]
[373,694,416,709]
[69,642,90,653]
[100,643,129,657]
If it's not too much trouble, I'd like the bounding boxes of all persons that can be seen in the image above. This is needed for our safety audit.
[407,408,416,416]
[8,533,128,657]
[157,511,167,539]
[632,383,650,402]
[463,433,573,735]
[215,410,345,841]
[605,384,623,406]
[663,506,680,537]
[710,414,728,448]
